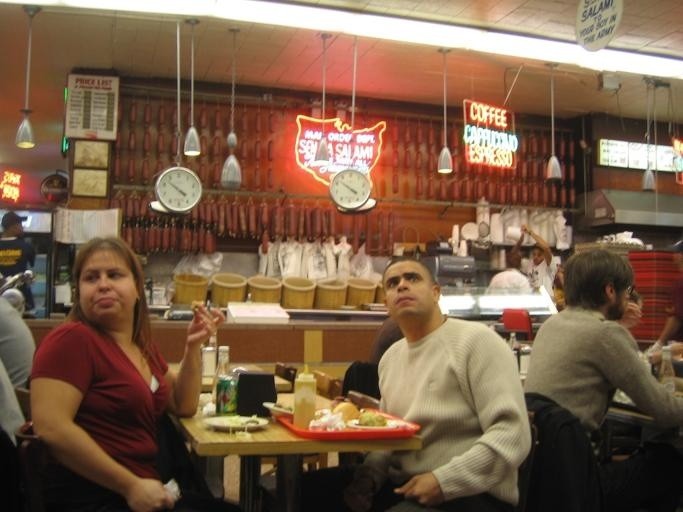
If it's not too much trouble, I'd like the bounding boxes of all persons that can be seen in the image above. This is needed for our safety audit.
[510,221,558,303]
[641,239,682,379]
[0,211,38,314]
[338,316,408,465]
[28,234,226,511]
[485,248,535,294]
[0,287,36,394]
[288,256,532,511]
[521,247,682,512]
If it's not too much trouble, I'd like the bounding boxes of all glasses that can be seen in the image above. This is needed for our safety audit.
[626,285,634,296]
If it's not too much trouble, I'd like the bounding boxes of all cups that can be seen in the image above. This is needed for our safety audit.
[170,273,388,310]
[499,249,506,269]
[450,223,468,259]
[489,205,570,251]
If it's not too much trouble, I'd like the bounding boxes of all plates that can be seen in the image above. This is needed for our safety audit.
[261,400,293,417]
[202,417,269,432]
[348,417,401,432]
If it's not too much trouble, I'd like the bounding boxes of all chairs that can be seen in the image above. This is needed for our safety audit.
[342,361,381,400]
[525,392,608,512]
[275,362,297,392]
[342,390,380,472]
[15,420,49,512]
[15,388,30,419]
[259,369,336,471]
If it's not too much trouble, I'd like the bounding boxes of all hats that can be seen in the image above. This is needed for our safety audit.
[1,211,28,228]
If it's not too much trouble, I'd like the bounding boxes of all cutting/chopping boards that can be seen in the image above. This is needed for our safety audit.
[199,373,293,393]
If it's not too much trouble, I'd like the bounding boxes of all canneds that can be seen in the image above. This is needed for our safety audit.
[214,376,238,417]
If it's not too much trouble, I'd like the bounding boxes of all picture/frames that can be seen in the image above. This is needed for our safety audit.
[68,137,115,199]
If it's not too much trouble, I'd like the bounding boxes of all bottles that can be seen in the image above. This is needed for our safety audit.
[201,347,216,376]
[507,332,518,353]
[293,364,317,430]
[217,344,231,378]
[659,346,676,398]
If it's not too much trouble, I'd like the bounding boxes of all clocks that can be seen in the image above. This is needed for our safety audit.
[156,166,203,213]
[330,169,372,209]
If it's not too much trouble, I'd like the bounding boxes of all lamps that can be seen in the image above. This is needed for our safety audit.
[641,78,655,191]
[437,48,453,174]
[14,4,42,149]
[545,62,563,181]
[184,18,242,189]
[315,33,332,165]
